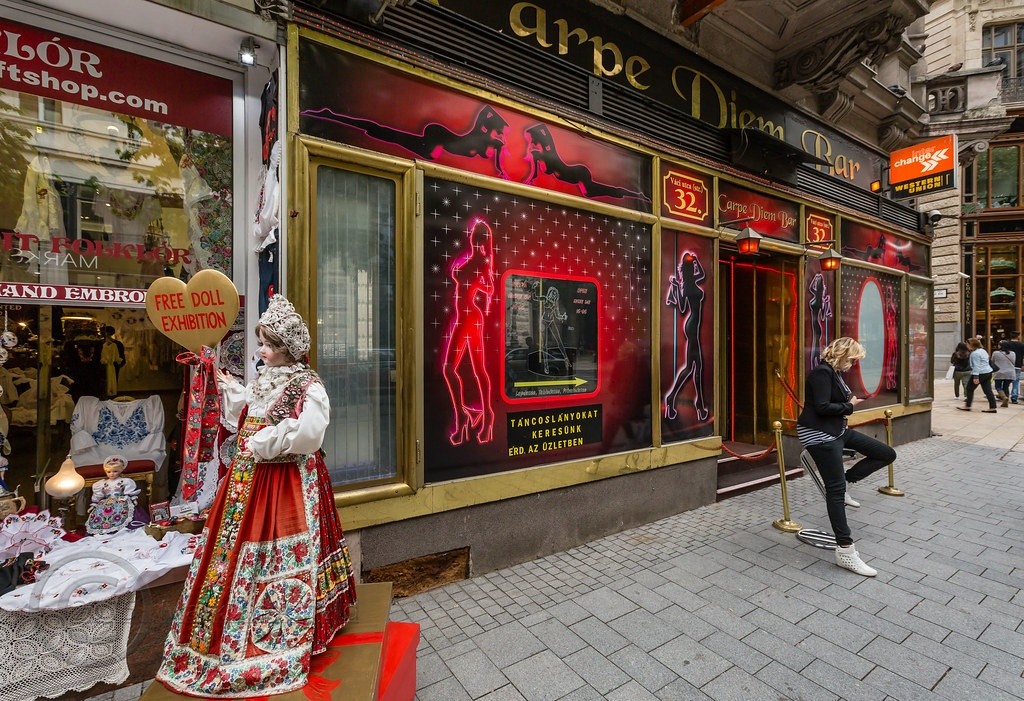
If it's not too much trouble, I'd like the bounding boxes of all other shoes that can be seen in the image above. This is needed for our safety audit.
[981,410,996,413]
[954,396,959,400]
[1011,397,1018,404]
[962,397,967,402]
[956,406,970,411]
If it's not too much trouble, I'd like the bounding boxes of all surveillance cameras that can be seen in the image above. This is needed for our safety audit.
[958,272,970,279]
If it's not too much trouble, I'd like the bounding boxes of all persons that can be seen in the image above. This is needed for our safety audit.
[92,326,125,401]
[155,285,357,697]
[12,148,163,288]
[797,337,896,576]
[951,331,1024,413]
[85,455,141,535]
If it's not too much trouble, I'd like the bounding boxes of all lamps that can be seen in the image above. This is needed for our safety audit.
[716,217,763,255]
[870,180,884,194]
[799,240,843,272]
[45,450,86,528]
[237,35,261,69]
[926,210,942,226]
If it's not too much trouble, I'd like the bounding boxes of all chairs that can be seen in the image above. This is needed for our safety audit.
[69,395,171,518]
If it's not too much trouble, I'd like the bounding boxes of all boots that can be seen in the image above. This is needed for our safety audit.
[1001,404,1008,407]
[998,390,1008,405]
[835,544,877,577]
[844,493,861,508]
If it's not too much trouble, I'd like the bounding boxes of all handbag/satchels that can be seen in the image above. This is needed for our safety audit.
[946,365,955,380]
[989,361,1000,373]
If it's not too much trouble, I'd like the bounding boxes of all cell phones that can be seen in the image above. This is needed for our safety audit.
[856,396,868,399]
